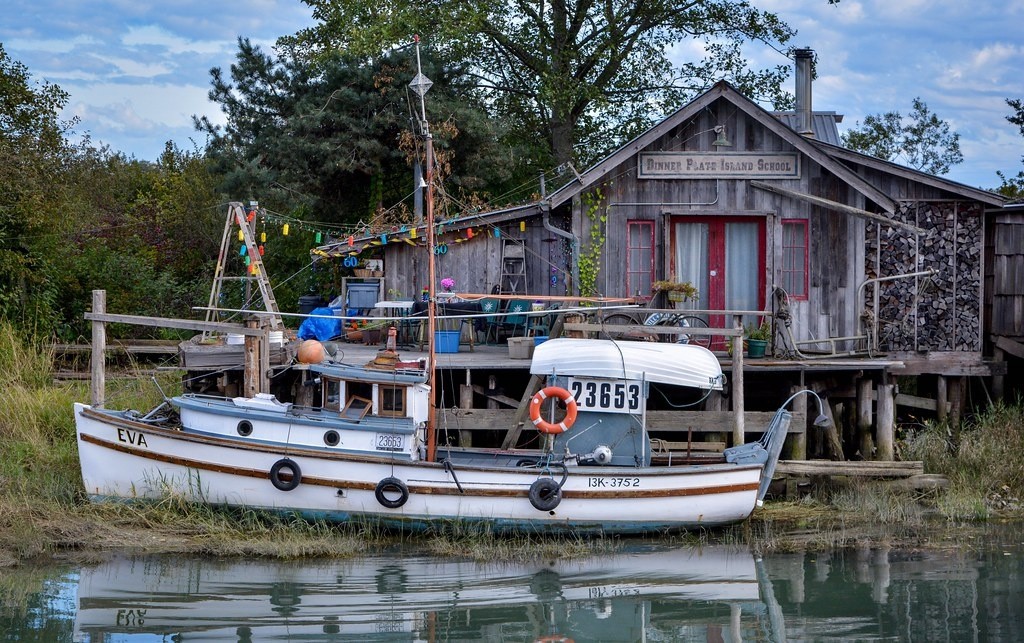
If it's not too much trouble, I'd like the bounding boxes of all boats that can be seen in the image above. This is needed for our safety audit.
[15,548,761,642]
[72,130,799,533]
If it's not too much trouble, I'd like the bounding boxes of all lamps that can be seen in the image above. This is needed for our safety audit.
[761,390,831,448]
[712,123,733,148]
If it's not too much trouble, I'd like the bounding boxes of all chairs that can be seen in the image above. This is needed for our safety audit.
[459,298,500,346]
[486,298,532,346]
[527,314,553,338]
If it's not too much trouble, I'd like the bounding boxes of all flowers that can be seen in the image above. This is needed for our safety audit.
[353,324,359,331]
[535,299,543,304]
[441,278,455,286]
[350,319,356,328]
[652,280,701,303]
[363,320,380,330]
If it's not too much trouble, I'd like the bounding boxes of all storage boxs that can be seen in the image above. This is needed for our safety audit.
[435,330,461,353]
[507,338,534,359]
[534,336,550,348]
[346,283,380,308]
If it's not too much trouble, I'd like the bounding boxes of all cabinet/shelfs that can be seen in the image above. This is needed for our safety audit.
[342,277,385,342]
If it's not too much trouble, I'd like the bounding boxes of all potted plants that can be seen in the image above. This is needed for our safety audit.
[353,265,359,277]
[372,265,384,277]
[199,338,222,346]
[747,322,770,357]
[359,263,372,278]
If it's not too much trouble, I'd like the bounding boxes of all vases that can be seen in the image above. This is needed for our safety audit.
[532,303,546,311]
[348,331,362,344]
[444,287,450,292]
[345,328,360,342]
[362,330,380,346]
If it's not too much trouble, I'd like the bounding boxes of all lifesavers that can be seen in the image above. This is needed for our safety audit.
[375,475,409,508]
[528,387,580,434]
[527,478,564,511]
[269,456,301,493]
